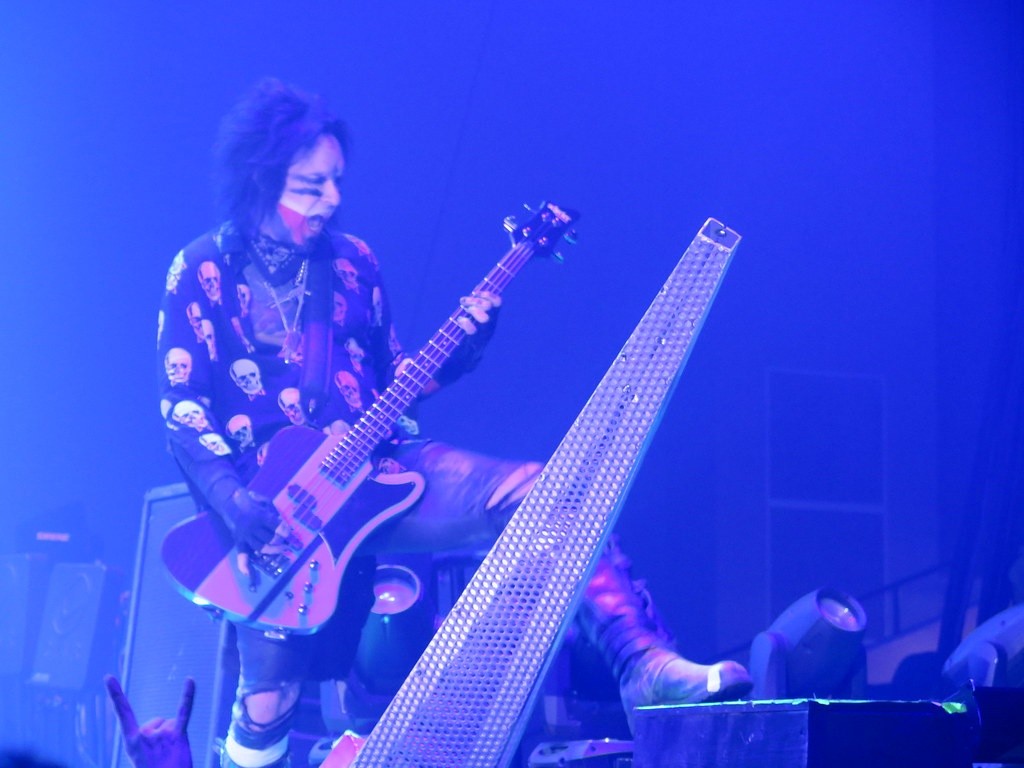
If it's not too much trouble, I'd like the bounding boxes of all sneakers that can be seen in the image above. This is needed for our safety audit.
[620,645,754,738]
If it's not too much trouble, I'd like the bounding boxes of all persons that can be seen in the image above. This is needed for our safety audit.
[157,78,755,767]
[0,675,196,767]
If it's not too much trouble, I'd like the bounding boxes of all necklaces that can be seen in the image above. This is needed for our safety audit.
[248,235,309,367]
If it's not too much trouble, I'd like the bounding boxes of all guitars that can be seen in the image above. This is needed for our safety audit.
[158,191,584,637]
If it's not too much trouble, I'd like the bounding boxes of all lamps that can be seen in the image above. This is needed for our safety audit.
[744,584,868,700]
